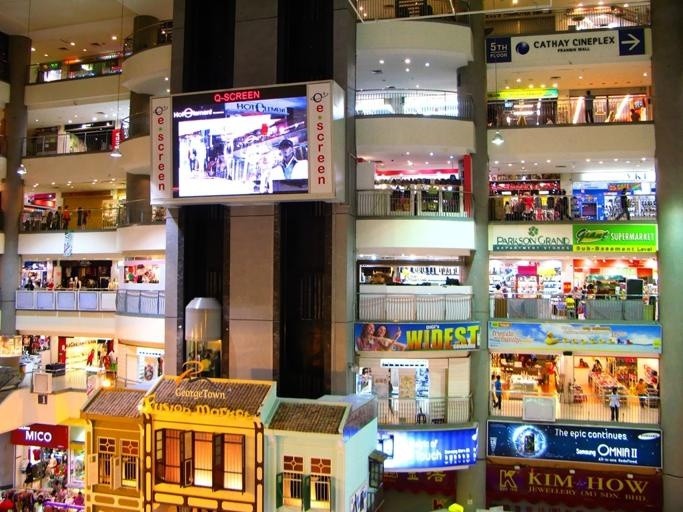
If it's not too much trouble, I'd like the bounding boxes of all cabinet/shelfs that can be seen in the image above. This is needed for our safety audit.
[489,272,565,305]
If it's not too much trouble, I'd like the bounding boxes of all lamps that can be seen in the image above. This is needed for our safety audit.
[108,0,123,157]
[491,62,505,147]
[17,0,31,174]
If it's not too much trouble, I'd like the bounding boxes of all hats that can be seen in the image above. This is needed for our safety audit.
[278,140,292,149]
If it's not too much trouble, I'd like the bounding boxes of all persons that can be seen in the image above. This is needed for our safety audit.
[614,188,631,221]
[71,491,85,511]
[635,377,646,409]
[47,205,88,230]
[630,108,642,121]
[490,373,498,406]
[188,147,199,175]
[270,139,310,195]
[500,352,538,368]
[493,375,501,410]
[493,285,504,300]
[592,359,602,373]
[609,388,621,422]
[26,462,33,489]
[356,324,401,351]
[503,189,573,221]
[374,325,406,351]
[86,348,119,371]
[583,89,597,123]
[565,280,609,319]
